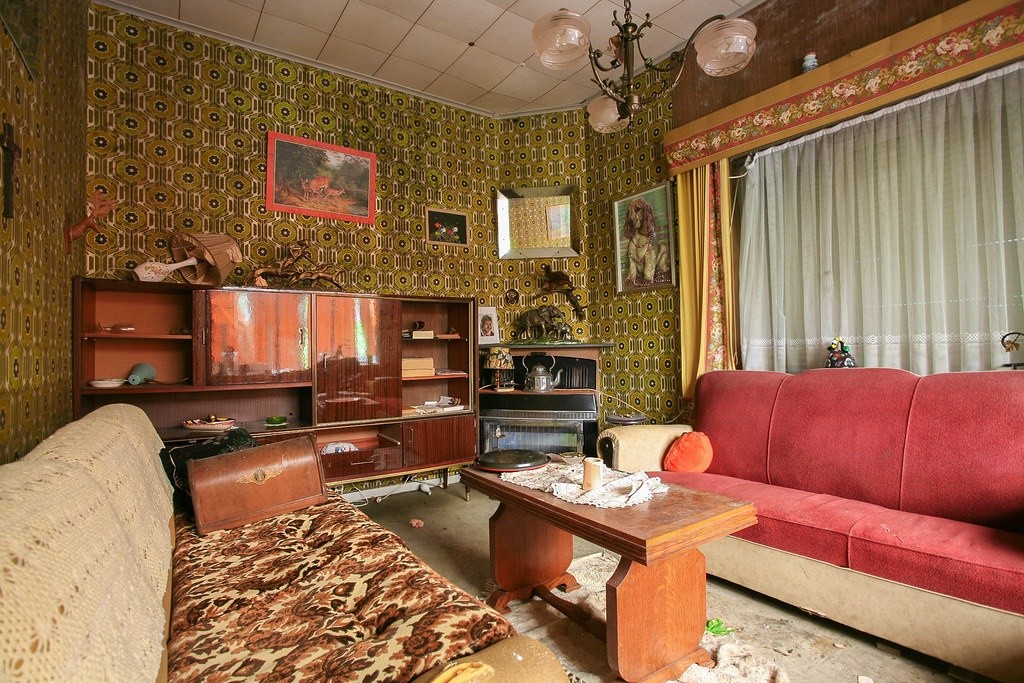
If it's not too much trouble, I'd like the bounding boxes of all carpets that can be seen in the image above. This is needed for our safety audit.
[478,549,787,683]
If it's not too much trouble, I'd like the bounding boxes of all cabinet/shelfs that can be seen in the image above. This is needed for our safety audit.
[65,272,480,502]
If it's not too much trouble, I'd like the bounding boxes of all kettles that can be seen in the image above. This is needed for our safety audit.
[522,351,564,391]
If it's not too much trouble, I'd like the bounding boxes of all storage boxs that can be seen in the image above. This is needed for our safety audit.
[402,356,434,369]
[402,368,436,377]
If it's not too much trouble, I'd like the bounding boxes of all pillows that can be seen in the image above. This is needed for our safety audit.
[661,430,714,475]
[160,428,260,507]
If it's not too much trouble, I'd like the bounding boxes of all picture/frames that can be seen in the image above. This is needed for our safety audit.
[478,306,500,344]
[496,183,582,260]
[424,206,470,249]
[266,129,377,225]
[613,180,680,296]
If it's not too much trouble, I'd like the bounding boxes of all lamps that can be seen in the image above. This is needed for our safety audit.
[484,346,516,392]
[132,230,244,284]
[530,0,758,135]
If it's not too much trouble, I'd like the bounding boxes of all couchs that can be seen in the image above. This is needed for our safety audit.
[595,366,1024,683]
[0,400,580,683]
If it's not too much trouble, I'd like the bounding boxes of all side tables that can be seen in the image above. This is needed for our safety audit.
[605,414,647,426]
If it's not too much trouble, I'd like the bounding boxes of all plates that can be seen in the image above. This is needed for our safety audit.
[182,417,236,431]
[89,380,127,388]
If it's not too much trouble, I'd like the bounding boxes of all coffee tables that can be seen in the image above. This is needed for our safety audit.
[452,450,762,683]
